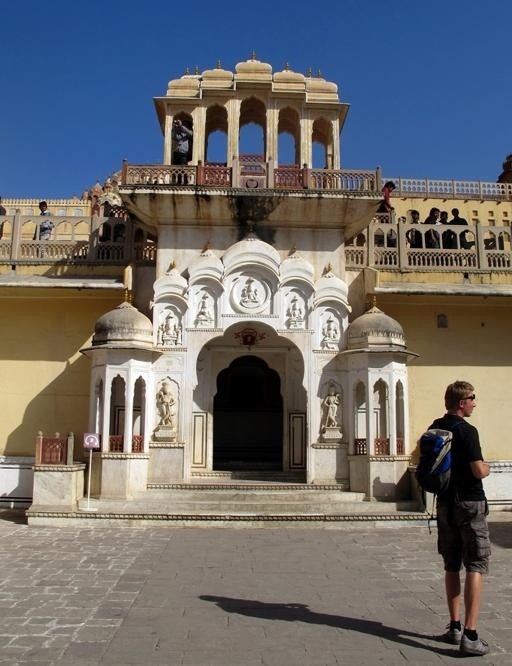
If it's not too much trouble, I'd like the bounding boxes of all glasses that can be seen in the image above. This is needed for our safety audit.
[461,394,475,400]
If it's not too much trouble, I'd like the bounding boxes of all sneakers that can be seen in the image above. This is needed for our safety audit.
[458,634,490,656]
[446,628,464,645]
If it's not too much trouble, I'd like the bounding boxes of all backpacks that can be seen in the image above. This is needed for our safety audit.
[413,418,467,495]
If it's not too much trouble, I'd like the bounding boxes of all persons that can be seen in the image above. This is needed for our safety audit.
[35,200,54,259]
[423,207,440,266]
[438,211,448,249]
[172,119,193,186]
[159,381,176,425]
[323,383,340,427]
[447,207,468,249]
[426,380,491,657]
[0,196,7,240]
[401,216,408,246]
[406,210,423,267]
[377,180,396,247]
